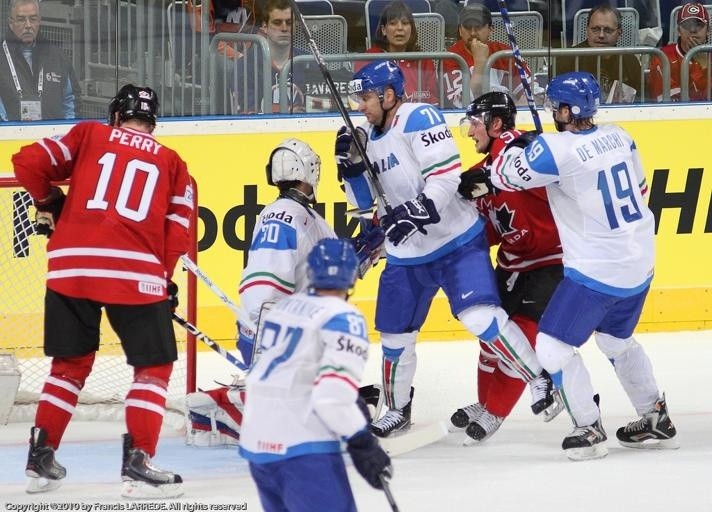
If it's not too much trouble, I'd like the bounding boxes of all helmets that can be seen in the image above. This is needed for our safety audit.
[109,84,159,129]
[347,59,406,100]
[465,91,517,116]
[309,237,359,290]
[266,138,321,203]
[546,70,601,120]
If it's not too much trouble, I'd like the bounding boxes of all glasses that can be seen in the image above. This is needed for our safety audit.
[8,15,41,25]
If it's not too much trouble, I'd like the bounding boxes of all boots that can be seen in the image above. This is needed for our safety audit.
[464,410,504,449]
[118,433,185,498]
[368,386,415,438]
[614,392,682,451]
[528,369,565,425]
[448,400,484,432]
[24,427,68,495]
[561,393,610,462]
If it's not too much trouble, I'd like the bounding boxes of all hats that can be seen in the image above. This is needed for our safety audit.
[678,3,709,25]
[459,2,492,28]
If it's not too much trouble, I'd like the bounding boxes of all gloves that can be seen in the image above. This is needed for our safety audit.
[351,216,387,280]
[333,126,369,177]
[456,170,501,202]
[34,186,66,238]
[350,436,395,489]
[381,192,441,245]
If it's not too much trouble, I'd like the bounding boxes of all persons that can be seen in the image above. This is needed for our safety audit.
[239,237,392,512]
[335,60,564,437]
[187,0,711,116]
[452,92,562,443]
[11,83,196,485]
[457,72,679,450]
[235,140,339,371]
[1,1,80,122]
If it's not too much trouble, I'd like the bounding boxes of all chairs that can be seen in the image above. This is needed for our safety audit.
[656,2,712,46]
[532,72,553,93]
[561,0,641,49]
[283,0,349,54]
[467,0,544,50]
[365,1,446,53]
